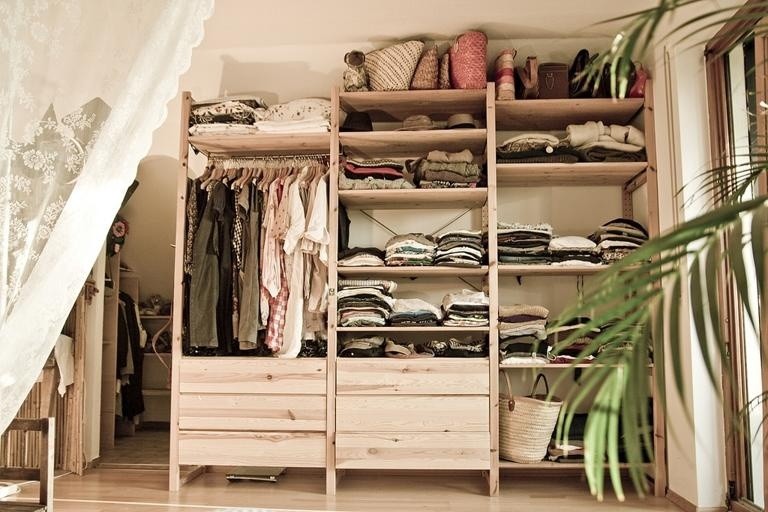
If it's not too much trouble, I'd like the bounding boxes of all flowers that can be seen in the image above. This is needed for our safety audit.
[106,214,130,259]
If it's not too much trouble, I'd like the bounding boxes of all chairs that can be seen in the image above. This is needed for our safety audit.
[0,418,54,512]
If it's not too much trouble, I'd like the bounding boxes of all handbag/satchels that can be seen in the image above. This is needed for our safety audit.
[364,31,648,100]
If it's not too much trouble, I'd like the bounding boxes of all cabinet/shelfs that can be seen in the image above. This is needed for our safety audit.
[491,79,668,498]
[138,315,172,432]
[75,240,121,464]
[168,88,335,497]
[331,81,496,496]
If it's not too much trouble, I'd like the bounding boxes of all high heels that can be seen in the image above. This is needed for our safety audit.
[139,294,162,315]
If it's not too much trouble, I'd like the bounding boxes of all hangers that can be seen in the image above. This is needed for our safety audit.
[199,152,330,190]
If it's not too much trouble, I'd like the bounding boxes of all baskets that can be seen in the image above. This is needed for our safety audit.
[499,368,563,465]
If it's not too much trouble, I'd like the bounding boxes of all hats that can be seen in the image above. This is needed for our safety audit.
[393,113,476,131]
[444,113,477,128]
[341,112,373,131]
